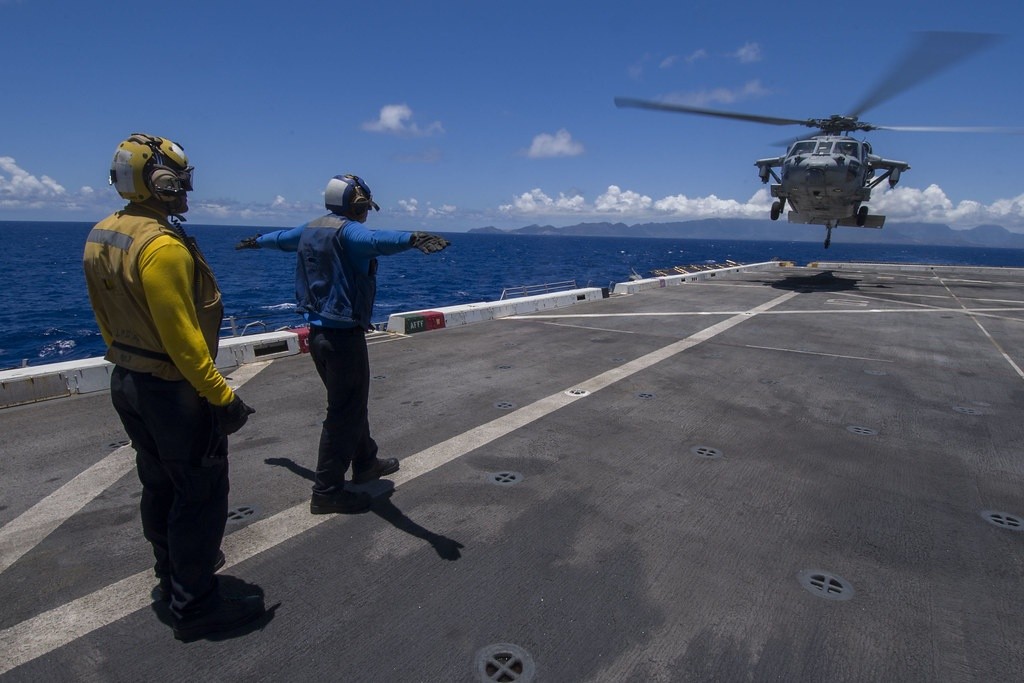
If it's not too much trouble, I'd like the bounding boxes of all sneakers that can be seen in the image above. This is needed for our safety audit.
[310,488,373,515]
[352,456,399,484]
[159,549,225,600]
[173,595,265,640]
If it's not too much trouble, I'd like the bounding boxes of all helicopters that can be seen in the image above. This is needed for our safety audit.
[614,28,1024,249]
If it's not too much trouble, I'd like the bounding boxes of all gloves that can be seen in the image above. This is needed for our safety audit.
[211,393,256,438]
[409,232,451,255]
[234,233,264,250]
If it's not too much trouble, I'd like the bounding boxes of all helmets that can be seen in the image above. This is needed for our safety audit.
[109,134,190,202]
[324,174,371,213]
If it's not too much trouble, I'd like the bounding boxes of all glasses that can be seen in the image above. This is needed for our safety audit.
[183,165,194,192]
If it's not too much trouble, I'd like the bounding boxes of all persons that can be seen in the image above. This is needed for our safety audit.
[83,134,265,639]
[236,173,451,514]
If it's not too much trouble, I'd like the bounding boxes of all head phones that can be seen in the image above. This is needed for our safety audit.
[344,174,370,218]
[128,133,183,205]
